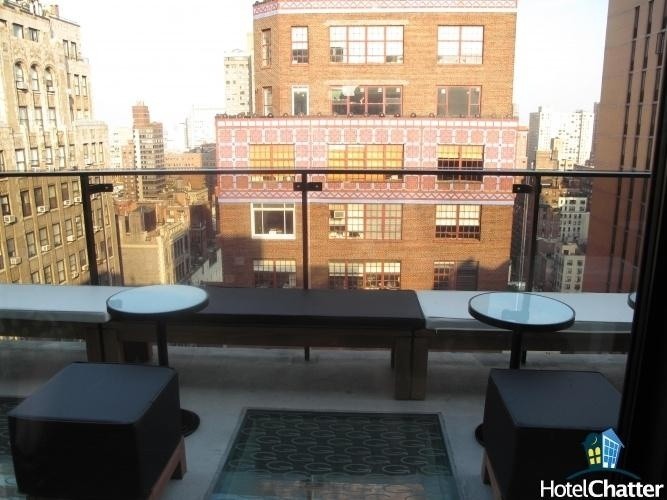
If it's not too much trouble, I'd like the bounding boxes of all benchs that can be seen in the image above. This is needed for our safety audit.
[0,284,636,403]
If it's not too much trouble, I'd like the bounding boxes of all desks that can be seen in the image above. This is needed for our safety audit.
[467,291,576,448]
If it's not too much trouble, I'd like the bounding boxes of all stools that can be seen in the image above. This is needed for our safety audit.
[474,369,622,499]
[8,362,183,500]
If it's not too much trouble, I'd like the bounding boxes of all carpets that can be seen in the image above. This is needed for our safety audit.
[202,404,472,500]
[0,396,25,500]
